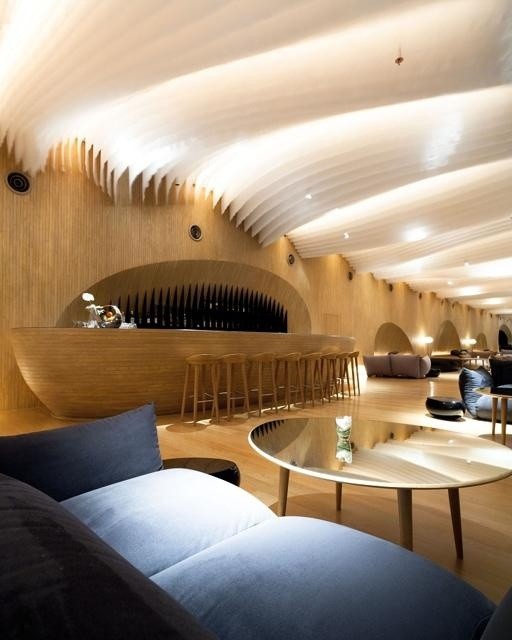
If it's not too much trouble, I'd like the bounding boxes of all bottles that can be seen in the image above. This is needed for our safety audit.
[122,312,126,323]
[130,309,135,323]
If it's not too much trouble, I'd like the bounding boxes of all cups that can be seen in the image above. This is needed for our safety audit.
[336,441,353,464]
[335,414,352,441]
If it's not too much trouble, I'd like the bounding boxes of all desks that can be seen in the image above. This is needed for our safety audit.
[472,387,512,445]
[248,417,512,559]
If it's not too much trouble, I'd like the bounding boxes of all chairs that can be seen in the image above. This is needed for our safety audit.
[362,350,512,422]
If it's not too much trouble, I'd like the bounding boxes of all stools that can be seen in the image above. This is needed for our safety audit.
[180,351,360,426]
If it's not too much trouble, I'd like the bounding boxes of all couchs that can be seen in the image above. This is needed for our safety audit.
[0,401,511,640]
